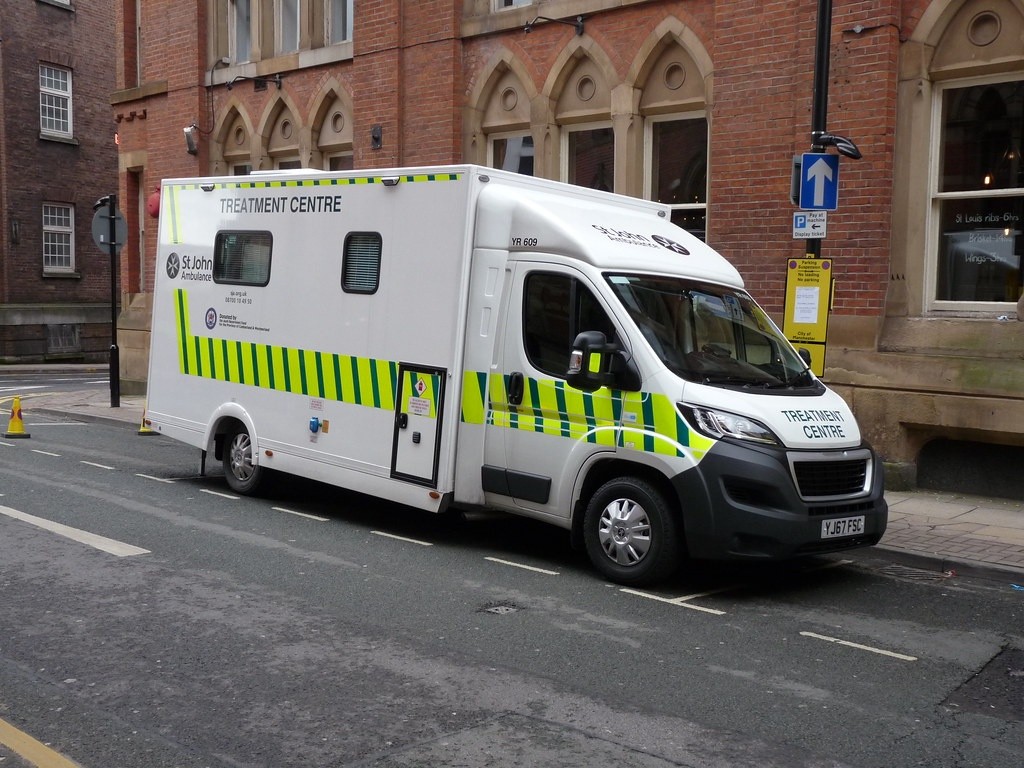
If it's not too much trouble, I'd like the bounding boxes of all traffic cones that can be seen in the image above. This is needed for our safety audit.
[138,395,160,435]
[2,396,31,438]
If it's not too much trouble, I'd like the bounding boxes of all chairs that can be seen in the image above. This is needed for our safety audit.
[539,296,623,390]
[636,292,683,368]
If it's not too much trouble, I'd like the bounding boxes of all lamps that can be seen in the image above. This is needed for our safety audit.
[225,76,281,91]
[524,16,584,36]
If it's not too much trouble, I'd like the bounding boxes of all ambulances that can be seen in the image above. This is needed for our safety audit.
[142,162,890,587]
